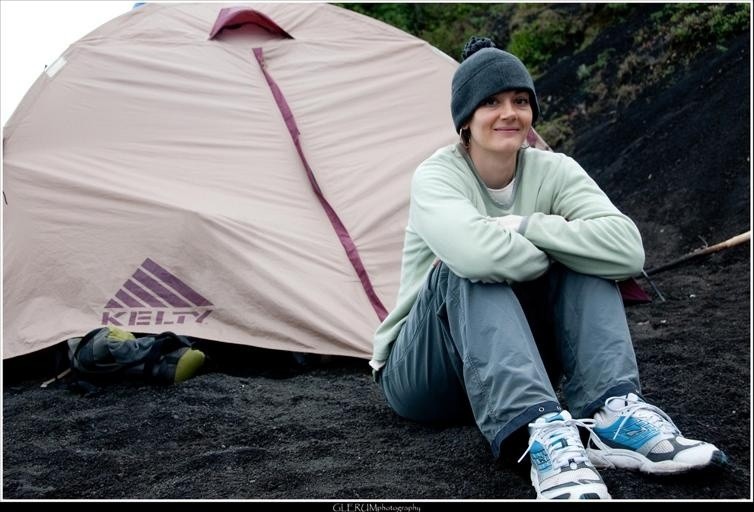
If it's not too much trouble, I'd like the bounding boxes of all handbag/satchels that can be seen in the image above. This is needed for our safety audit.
[71,328,205,387]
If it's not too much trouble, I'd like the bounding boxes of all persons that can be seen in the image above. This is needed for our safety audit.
[366,32,730,498]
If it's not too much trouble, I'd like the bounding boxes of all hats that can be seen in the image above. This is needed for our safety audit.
[450,36,540,135]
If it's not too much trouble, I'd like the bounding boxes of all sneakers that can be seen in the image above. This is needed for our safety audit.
[586,392,729,476]
[529,409,613,501]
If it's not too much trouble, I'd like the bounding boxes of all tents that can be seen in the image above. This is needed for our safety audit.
[4,3,559,381]
